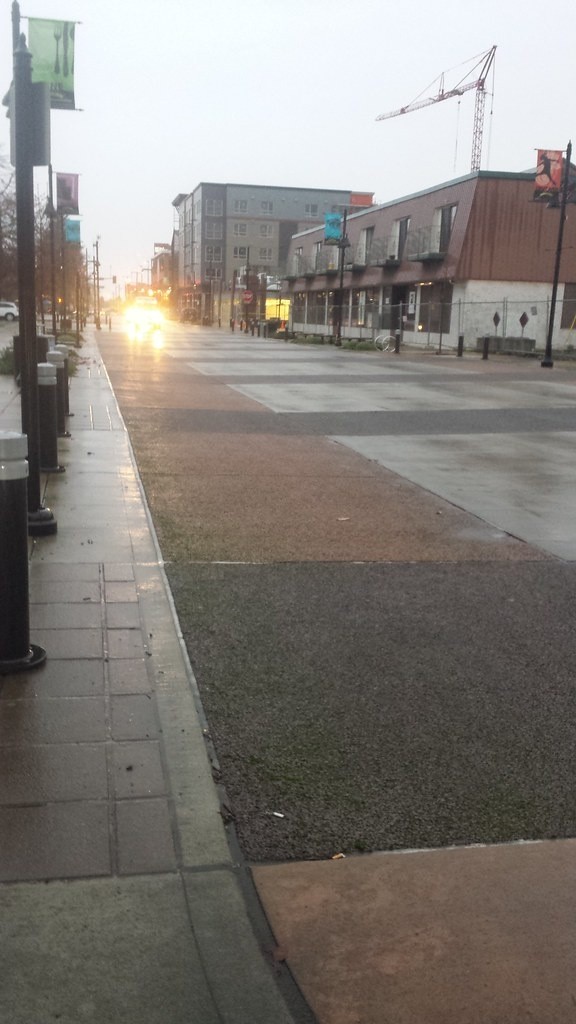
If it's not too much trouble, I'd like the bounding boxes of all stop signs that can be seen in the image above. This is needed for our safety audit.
[243,291,253,304]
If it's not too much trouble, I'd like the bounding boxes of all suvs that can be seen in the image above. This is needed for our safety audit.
[0,302,19,321]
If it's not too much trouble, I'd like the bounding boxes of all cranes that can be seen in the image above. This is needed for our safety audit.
[374,45,498,173]
